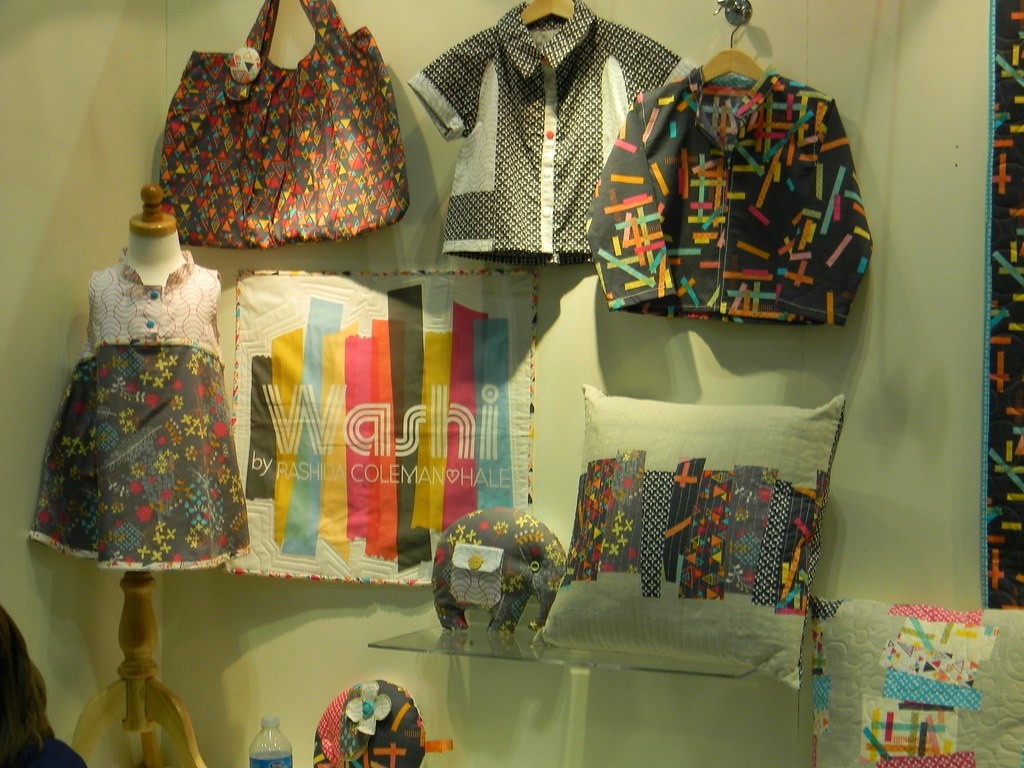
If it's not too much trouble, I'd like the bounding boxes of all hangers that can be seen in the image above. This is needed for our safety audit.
[702,3,764,84]
[521,0,576,27]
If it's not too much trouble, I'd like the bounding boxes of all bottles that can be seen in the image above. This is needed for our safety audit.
[250,715,292,768]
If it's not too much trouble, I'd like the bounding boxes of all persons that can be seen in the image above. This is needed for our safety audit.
[30,185,252,571]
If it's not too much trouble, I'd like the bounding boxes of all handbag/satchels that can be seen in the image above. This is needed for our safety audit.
[159,1,410,252]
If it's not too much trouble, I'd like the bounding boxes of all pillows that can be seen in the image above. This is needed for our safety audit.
[808,594,1024,768]
[530,382,846,690]
